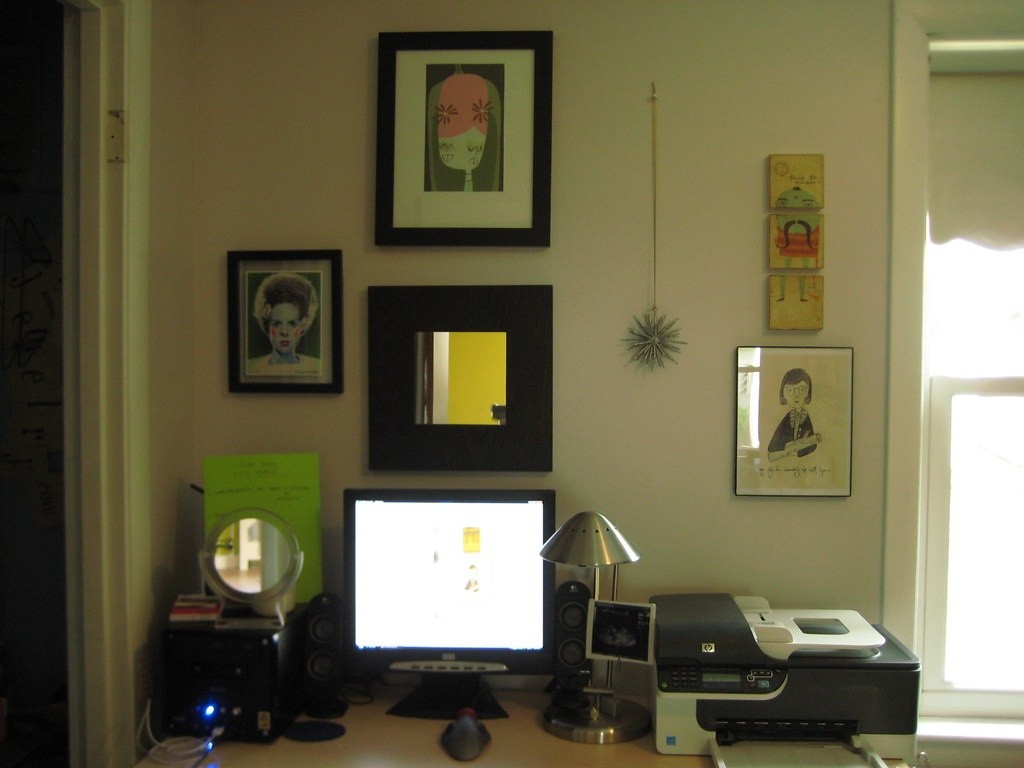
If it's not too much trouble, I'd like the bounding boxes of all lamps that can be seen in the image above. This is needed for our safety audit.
[539,511,651,745]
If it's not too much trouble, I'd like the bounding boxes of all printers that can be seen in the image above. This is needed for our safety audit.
[647,592,921,768]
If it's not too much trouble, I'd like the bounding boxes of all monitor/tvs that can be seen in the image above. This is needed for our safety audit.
[340,487,558,721]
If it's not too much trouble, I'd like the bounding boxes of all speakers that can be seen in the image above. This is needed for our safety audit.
[300,591,350,719]
[543,581,595,720]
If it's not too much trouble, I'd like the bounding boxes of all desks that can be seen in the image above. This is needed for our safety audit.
[134,688,907,768]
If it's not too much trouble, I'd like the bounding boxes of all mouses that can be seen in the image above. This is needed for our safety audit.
[440,708,490,761]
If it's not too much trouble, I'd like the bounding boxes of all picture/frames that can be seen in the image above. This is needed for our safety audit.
[368,285,554,473]
[373,30,554,248]
[226,249,344,395]
[735,346,854,498]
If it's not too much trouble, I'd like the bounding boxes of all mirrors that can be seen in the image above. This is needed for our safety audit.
[201,508,304,630]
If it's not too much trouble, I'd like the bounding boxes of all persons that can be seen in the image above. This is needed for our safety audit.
[252,273,320,372]
